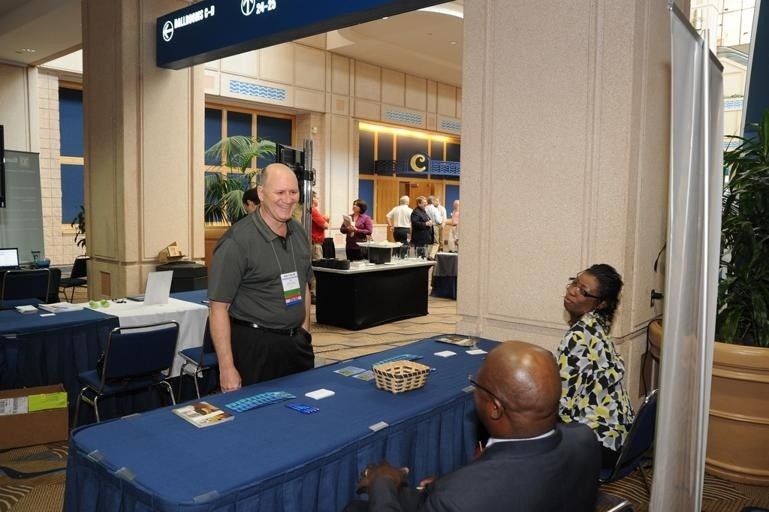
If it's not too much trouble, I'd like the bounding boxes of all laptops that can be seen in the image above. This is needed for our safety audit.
[127,270,173,306]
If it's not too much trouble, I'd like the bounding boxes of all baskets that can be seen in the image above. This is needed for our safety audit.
[371,360,431,394]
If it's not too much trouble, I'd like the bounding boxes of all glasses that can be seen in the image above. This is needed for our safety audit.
[466,375,506,411]
[568,277,599,299]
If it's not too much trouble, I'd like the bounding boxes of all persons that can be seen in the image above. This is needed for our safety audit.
[205,161,316,394]
[551,264,636,469]
[309,189,329,262]
[242,186,260,216]
[341,199,374,260]
[353,338,608,511]
[426,197,441,258]
[434,199,447,250]
[386,195,414,259]
[411,197,434,246]
[448,199,459,249]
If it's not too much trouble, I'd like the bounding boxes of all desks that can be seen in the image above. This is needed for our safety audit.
[311,239,437,330]
[61,332,529,512]
[430,249,460,304]
[1,288,212,382]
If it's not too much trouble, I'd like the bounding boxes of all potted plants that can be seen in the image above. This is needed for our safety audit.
[648,114,769,487]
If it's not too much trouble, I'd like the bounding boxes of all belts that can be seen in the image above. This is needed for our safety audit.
[229,315,300,336]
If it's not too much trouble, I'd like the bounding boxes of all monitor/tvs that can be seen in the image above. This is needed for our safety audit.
[276,142,304,204]
[0,248,19,268]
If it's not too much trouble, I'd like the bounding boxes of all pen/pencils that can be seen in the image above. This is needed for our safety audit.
[201,300,210,304]
[113,297,125,304]
[286,401,320,415]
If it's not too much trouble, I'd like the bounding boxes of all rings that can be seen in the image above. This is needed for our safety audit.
[364,468,367,474]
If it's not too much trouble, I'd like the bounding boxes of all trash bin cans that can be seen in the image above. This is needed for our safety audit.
[156,261,207,293]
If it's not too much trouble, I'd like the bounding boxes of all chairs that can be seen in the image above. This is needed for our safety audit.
[591,373,661,496]
[4,266,50,303]
[178,315,223,400]
[60,254,90,302]
[70,319,186,428]
[323,236,336,257]
